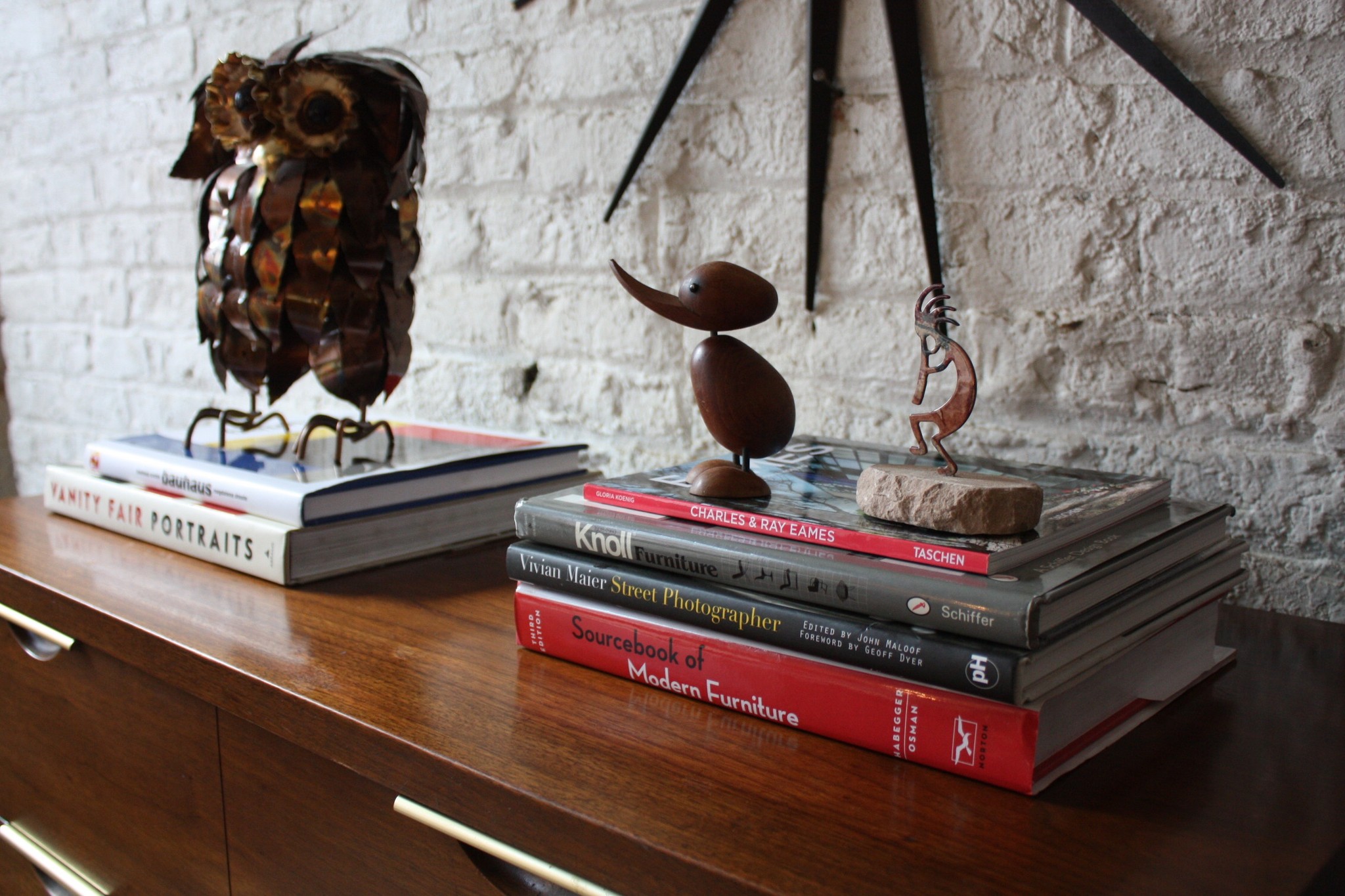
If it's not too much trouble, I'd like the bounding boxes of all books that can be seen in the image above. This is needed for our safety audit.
[507,436,1246,796]
[40,420,601,588]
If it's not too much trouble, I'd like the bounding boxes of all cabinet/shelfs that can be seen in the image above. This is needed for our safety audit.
[0,494,1344,896]
